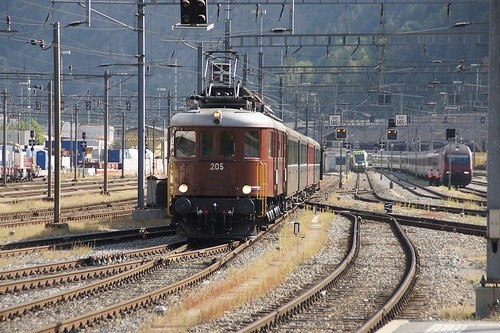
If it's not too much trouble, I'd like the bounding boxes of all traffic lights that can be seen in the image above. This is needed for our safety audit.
[335,127,349,140]
[385,129,398,141]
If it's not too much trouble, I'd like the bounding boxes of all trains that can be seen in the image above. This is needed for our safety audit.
[370,142,473,188]
[350,148,369,173]
[164,48,327,248]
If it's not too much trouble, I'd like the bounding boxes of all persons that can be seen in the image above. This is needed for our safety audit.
[428,169,434,185]
[436,169,441,187]
